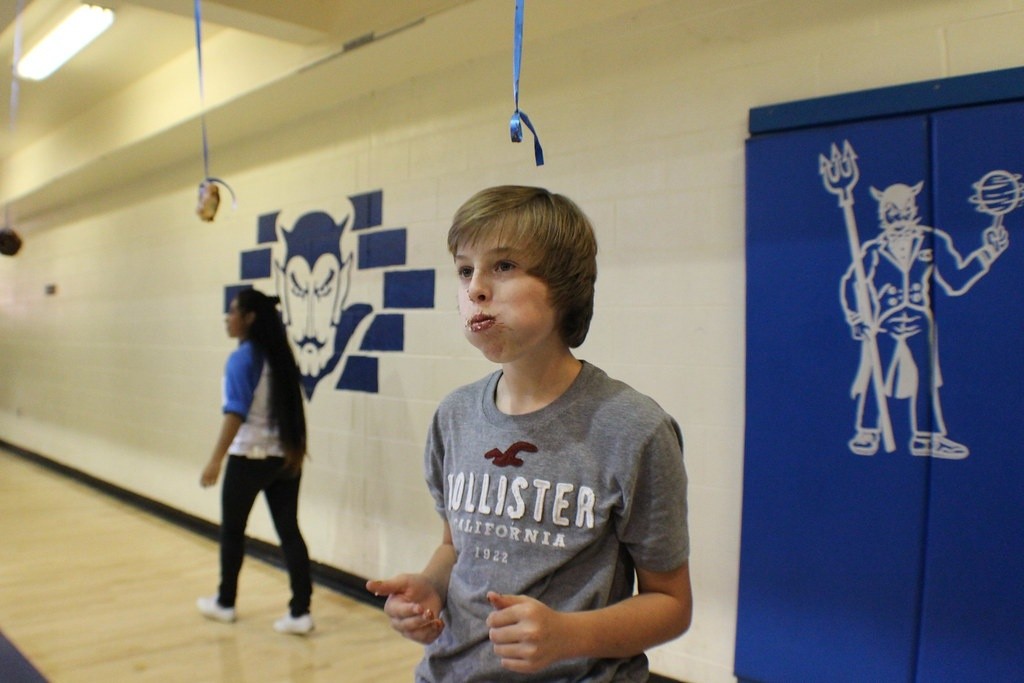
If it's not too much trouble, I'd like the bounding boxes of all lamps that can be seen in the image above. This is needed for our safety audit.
[16,4,113,81]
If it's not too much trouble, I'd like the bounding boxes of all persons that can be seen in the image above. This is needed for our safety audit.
[194,288,316,639]
[367,185,696,683]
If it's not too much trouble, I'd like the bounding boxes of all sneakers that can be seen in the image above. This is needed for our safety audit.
[198,595,236,622]
[274,615,315,636]
